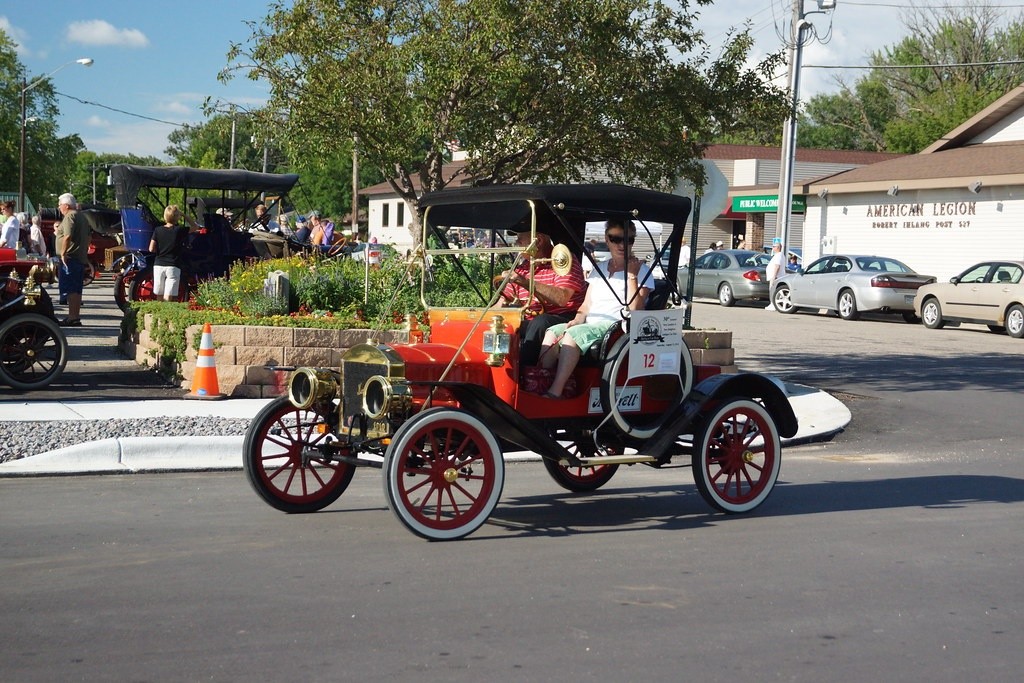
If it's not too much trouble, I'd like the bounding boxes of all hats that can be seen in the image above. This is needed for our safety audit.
[297,215,305,223]
[791,255,797,260]
[716,241,722,247]
[507,218,551,236]
[681,236,687,243]
[308,210,321,219]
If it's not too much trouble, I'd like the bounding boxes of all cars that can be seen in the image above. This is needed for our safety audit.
[913,260,1024,338]
[646,247,671,272]
[110,162,305,315]
[0,248,71,392]
[582,239,613,272]
[770,254,941,325]
[244,182,799,542]
[352,242,389,264]
[673,250,796,307]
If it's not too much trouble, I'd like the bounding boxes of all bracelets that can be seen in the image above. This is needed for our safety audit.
[629,276,637,280]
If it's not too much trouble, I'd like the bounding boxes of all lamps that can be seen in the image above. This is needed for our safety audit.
[817,188,829,201]
[887,185,899,197]
[966,180,983,194]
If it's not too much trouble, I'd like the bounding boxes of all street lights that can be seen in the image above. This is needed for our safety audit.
[19,57,94,211]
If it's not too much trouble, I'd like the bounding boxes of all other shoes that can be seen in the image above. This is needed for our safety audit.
[766,303,776,312]
[60,299,67,304]
[541,392,561,401]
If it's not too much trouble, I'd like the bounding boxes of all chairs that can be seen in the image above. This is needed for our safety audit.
[835,265,849,272]
[996,271,1013,283]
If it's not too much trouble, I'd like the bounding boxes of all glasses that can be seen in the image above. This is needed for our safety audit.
[607,234,635,246]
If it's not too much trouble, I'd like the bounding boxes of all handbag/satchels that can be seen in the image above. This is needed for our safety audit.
[519,337,577,400]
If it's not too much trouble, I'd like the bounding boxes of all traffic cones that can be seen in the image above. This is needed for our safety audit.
[181,322,233,401]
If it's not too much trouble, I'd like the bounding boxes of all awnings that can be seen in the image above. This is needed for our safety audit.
[716,197,747,220]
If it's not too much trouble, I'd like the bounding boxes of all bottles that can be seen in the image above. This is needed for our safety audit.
[15,241,26,260]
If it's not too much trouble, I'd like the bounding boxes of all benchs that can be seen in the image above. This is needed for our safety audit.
[578,278,670,396]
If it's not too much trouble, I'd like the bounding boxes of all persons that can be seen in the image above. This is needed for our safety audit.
[15,212,61,258]
[738,240,746,249]
[583,239,597,280]
[250,205,280,232]
[492,211,587,390]
[536,215,655,398]
[216,208,233,218]
[149,205,198,302]
[765,242,785,311]
[703,241,724,254]
[677,236,691,300]
[56,193,90,327]
[280,210,343,245]
[788,255,801,271]
[0,199,20,249]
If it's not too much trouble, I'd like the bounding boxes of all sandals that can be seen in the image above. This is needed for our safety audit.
[58,317,82,327]
